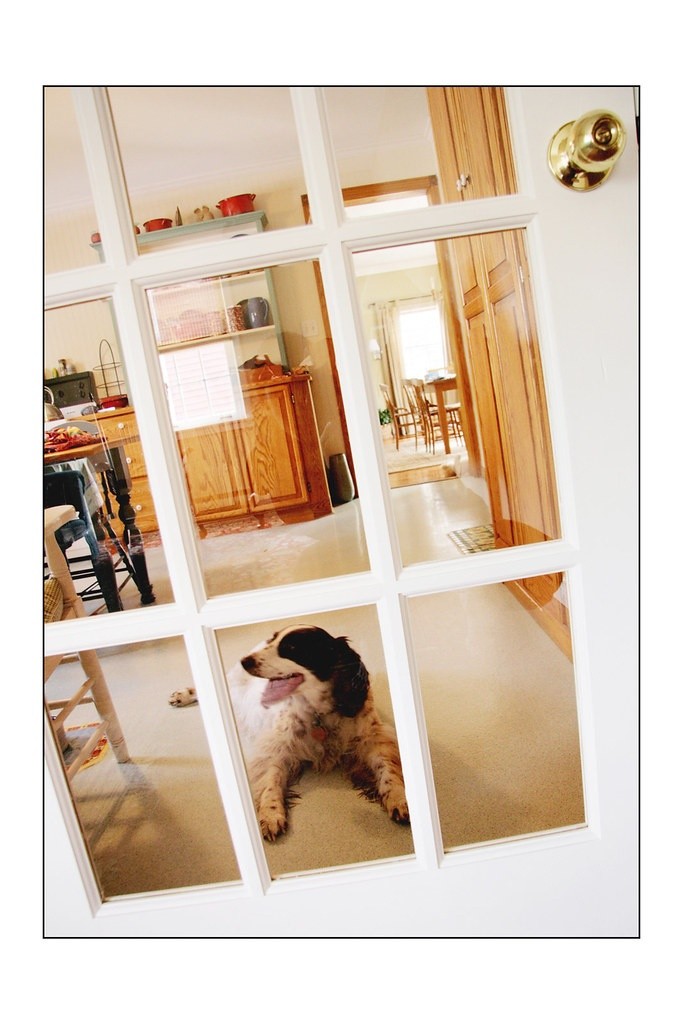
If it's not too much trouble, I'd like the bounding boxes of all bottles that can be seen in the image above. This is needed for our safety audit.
[58,359,67,376]
[328,453,355,503]
[238,297,269,329]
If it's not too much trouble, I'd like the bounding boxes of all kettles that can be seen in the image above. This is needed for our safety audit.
[44,386,64,422]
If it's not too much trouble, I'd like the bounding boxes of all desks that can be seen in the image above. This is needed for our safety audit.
[43,431,156,613]
[423,375,458,454]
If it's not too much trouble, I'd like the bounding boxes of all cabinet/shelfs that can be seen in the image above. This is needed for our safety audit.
[425,86,572,661]
[90,210,339,548]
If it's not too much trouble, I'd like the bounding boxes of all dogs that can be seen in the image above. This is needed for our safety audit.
[169,624,410,842]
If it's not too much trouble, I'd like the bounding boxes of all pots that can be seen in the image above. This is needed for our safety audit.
[143,218,173,232]
[215,193,256,216]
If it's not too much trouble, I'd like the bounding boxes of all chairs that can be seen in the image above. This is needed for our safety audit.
[399,378,462,455]
[379,383,427,450]
[43,505,129,784]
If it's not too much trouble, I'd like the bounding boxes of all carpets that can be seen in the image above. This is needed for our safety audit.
[448,522,495,555]
